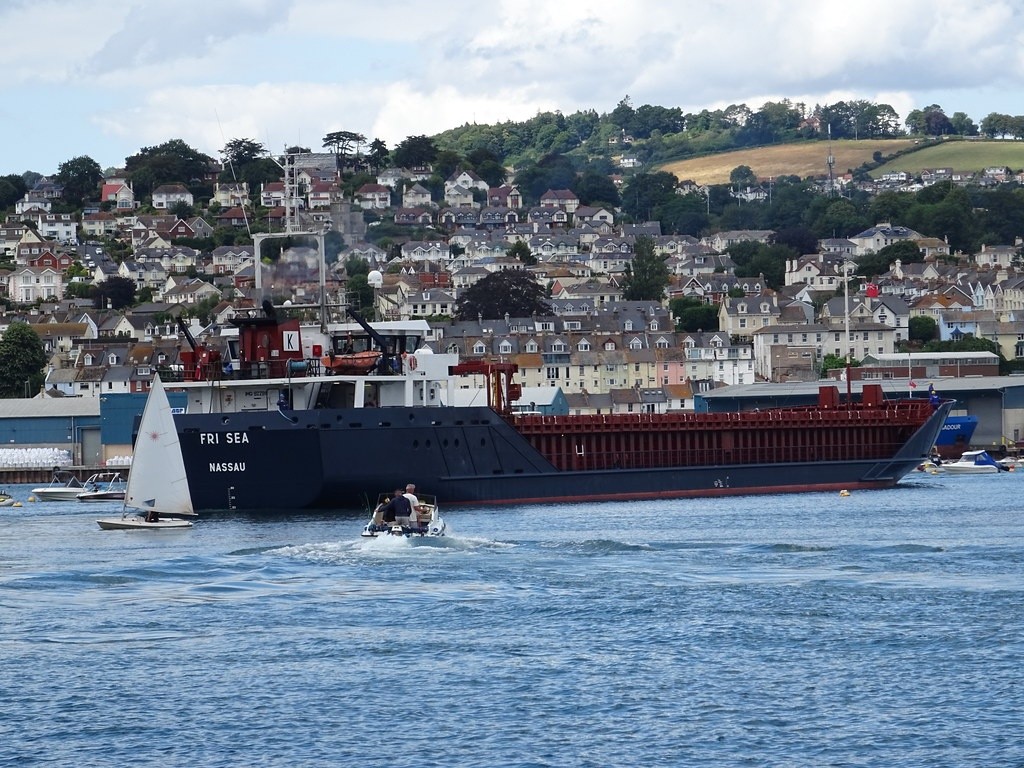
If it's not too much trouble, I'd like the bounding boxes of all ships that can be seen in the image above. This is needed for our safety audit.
[133,230,957,512]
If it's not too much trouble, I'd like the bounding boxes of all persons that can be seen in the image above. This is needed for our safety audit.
[928,390,942,409]
[403,484,427,528]
[374,489,412,526]
[929,382,934,397]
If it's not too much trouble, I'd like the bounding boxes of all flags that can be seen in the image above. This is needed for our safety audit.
[909,381,916,389]
[864,276,879,297]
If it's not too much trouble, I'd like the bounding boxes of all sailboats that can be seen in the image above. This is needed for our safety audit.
[97,369,198,530]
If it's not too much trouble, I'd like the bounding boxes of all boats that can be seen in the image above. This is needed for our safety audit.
[995,456,1022,468]
[941,448,1002,475]
[930,415,979,460]
[77,473,127,503]
[918,457,948,473]
[360,492,446,540]
[0,491,14,507]
[30,470,88,502]
